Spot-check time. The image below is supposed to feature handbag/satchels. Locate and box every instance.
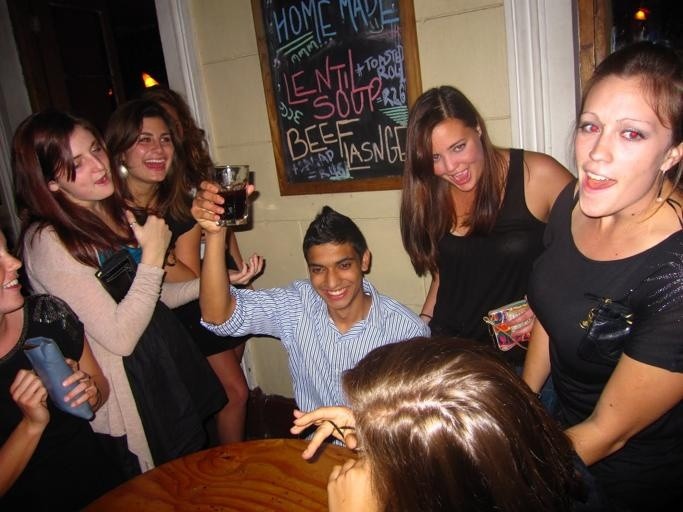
[574,289,637,369]
[23,337,96,422]
[95,247,139,304]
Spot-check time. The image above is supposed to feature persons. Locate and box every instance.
[0,228,125,512]
[104,97,202,285]
[188,180,432,453]
[9,106,264,475]
[399,85,576,378]
[517,39,683,512]
[290,337,601,512]
[143,83,257,443]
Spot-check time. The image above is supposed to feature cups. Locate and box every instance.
[210,164,253,229]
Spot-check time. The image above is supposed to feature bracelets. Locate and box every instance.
[419,313,433,320]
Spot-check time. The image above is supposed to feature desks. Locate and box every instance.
[77,436,361,512]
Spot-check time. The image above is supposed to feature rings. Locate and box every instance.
[129,222,137,228]
